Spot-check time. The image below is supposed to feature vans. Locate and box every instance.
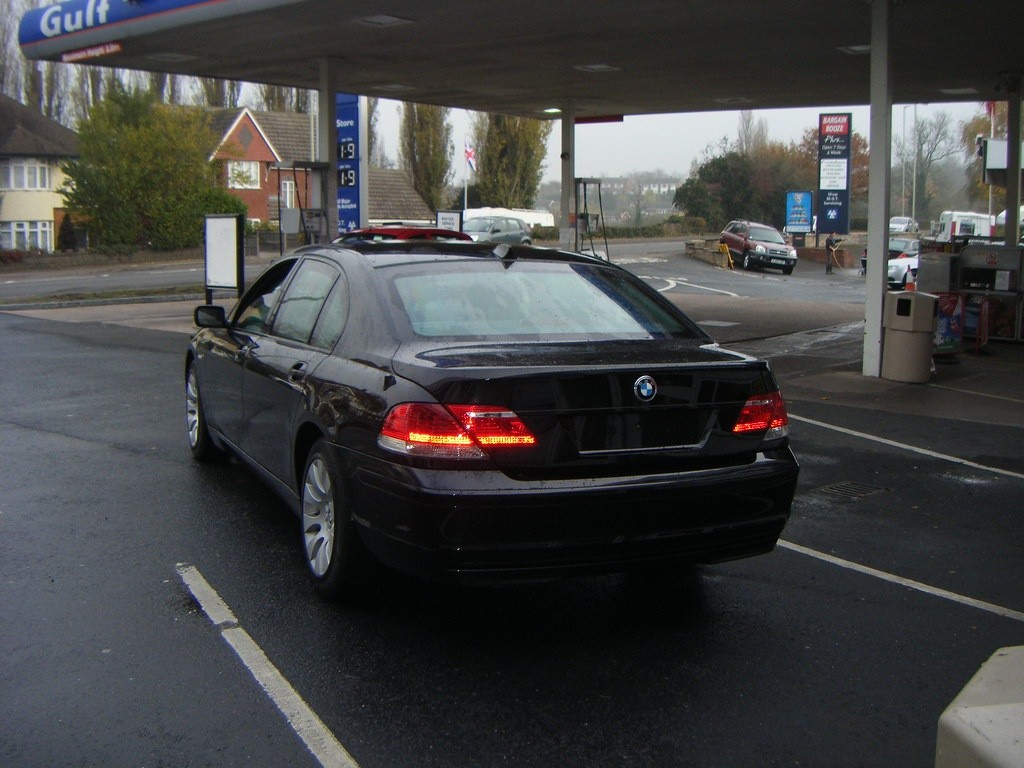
[938,210,996,244]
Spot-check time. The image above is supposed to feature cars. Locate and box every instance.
[888,217,920,235]
[179,237,803,613]
[861,235,921,277]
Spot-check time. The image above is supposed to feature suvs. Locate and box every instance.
[721,219,799,277]
[887,253,918,288]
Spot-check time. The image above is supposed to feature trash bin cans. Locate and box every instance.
[881,291,939,384]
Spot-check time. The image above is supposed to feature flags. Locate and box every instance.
[464,139,482,172]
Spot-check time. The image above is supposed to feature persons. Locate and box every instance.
[825,230,845,275]
